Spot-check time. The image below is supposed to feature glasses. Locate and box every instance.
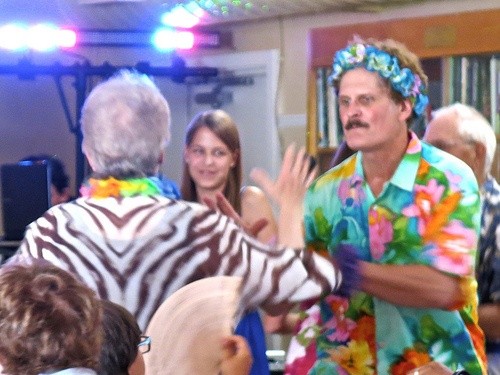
[136,336,151,354]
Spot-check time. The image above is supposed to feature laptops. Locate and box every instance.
[0,161,51,241]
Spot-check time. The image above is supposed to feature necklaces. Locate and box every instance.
[81,177,180,198]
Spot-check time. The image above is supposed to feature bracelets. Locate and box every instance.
[337,247,357,297]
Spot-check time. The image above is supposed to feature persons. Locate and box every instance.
[182,110,280,250]
[264,141,358,375]
[0,151,253,375]
[423,103,500,375]
[10,73,341,333]
[204,39,488,375]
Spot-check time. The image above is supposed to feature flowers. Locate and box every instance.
[327,42,428,117]
